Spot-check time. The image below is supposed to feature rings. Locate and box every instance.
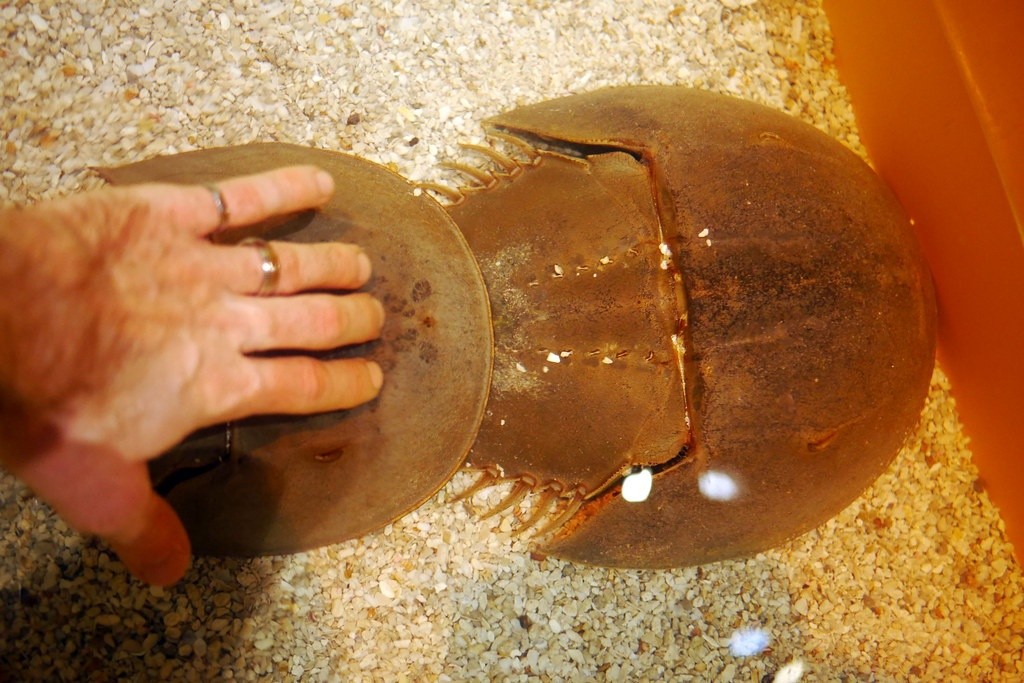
[238,237,279,296]
[202,183,226,232]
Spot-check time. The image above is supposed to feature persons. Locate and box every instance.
[0,163,386,588]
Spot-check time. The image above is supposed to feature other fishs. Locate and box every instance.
[87,144,495,560]
[414,84,930,571]
